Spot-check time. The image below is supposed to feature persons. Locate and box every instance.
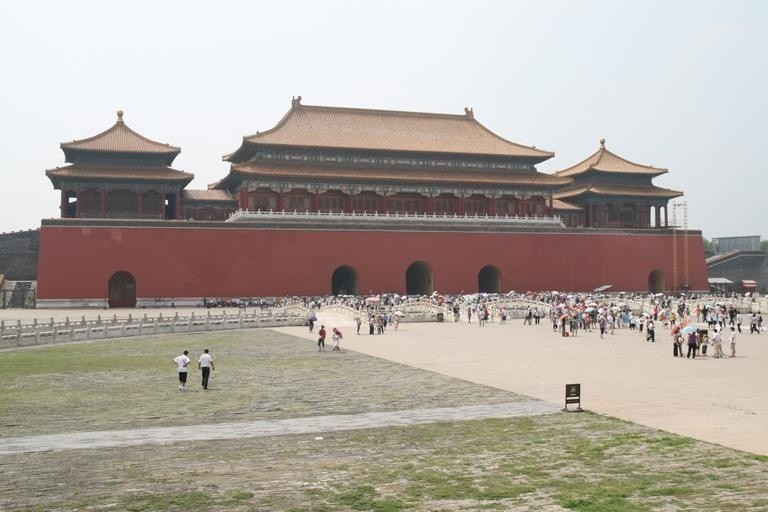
[408,290,635,340]
[325,292,402,335]
[638,290,764,358]
[198,349,214,390]
[318,326,326,350]
[205,294,324,309]
[172,350,190,391]
[332,328,343,350]
[171,297,176,309]
[307,309,316,331]
